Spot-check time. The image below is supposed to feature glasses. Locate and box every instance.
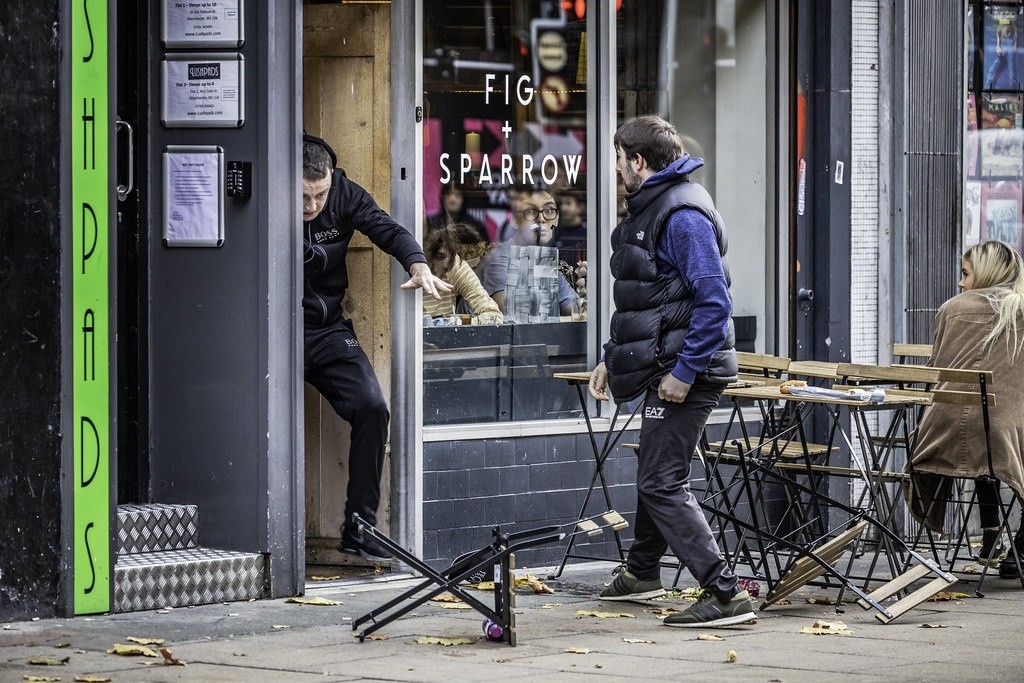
[513,207,560,221]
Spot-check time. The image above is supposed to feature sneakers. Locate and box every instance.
[340,523,395,562]
[662,587,758,628]
[598,564,667,601]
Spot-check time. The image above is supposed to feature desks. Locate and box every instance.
[551,358,928,605]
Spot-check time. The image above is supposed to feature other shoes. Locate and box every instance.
[999,559,1024,579]
[979,541,1008,564]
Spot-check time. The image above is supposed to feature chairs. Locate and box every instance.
[346,507,628,646]
[621,341,1024,625]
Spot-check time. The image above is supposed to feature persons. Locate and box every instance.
[586,115,758,628]
[549,188,587,266]
[456,180,587,320]
[901,240,1024,580]
[496,182,543,246]
[422,190,504,325]
[426,179,492,244]
[301,134,453,562]
[986,6,1020,89]
